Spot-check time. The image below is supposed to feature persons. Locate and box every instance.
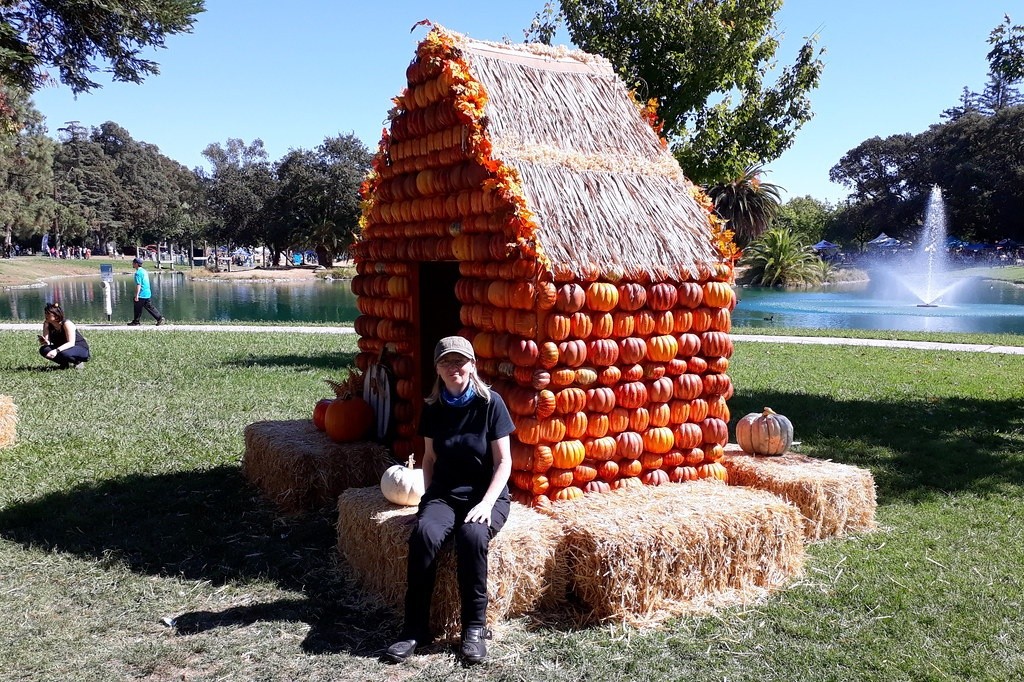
[36,302,91,370]
[0,241,92,260]
[383,335,517,666]
[127,258,165,326]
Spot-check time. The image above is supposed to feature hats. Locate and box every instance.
[435,336,475,365]
[131,258,144,266]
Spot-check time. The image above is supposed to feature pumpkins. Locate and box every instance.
[314,393,377,441]
[735,406,794,456]
[348,38,737,506]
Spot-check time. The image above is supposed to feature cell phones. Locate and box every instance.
[37,335,48,345]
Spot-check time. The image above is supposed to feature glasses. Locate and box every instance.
[437,361,471,367]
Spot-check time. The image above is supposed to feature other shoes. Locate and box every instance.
[127,320,140,326]
[459,625,488,661]
[60,361,85,370]
[156,317,165,326]
[386,637,429,663]
